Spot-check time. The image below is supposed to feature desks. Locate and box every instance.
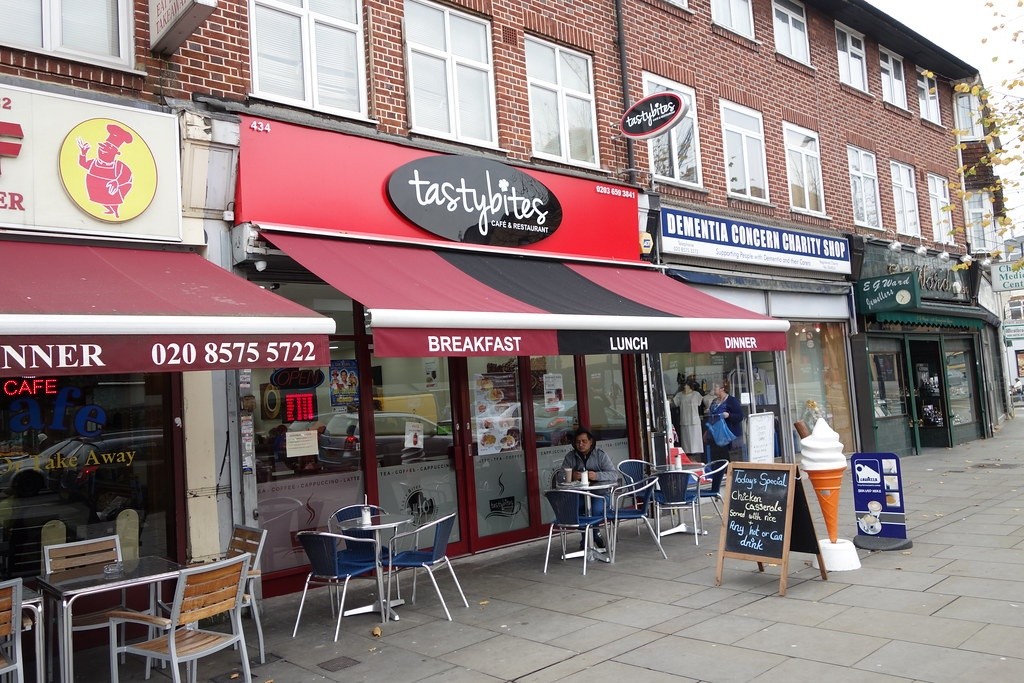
[650,463,707,537]
[35,555,188,683]
[22,585,44,683]
[557,480,616,562]
[335,513,414,622]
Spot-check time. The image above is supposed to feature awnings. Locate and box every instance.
[258,232,790,357]
[0,242,336,379]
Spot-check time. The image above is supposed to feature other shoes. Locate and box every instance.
[594,530,606,548]
[580,540,584,550]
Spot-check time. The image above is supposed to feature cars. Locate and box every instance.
[45,429,164,495]
[66,432,167,505]
[318,411,456,468]
[469,402,539,451]
[0,434,80,497]
[280,412,345,470]
[946,370,966,387]
[532,400,625,447]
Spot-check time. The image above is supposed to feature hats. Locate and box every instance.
[717,379,730,393]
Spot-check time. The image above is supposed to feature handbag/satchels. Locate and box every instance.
[705,413,737,447]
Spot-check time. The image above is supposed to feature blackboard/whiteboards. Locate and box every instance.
[747,412,775,462]
[717,461,823,564]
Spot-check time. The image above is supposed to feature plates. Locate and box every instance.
[479,380,516,450]
[859,518,882,534]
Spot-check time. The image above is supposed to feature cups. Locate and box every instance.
[563,468,572,483]
[867,501,882,520]
[866,515,877,530]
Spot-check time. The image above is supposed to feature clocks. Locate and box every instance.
[895,289,912,305]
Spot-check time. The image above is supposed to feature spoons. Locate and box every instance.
[858,518,868,532]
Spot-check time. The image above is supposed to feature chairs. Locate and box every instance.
[651,469,703,546]
[166,524,268,665]
[292,530,385,638]
[43,535,125,683]
[616,460,654,535]
[687,459,729,523]
[542,488,612,575]
[609,475,668,563]
[385,512,469,622]
[101,552,255,683]
[0,577,24,683]
[550,469,567,552]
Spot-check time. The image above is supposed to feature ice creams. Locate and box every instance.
[793,418,848,543]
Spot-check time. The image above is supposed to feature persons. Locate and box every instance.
[330,371,358,389]
[589,386,612,427]
[673,383,717,454]
[703,380,744,500]
[557,428,618,551]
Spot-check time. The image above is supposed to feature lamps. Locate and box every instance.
[928,240,950,262]
[974,249,993,270]
[904,234,928,257]
[949,242,973,265]
[862,228,902,253]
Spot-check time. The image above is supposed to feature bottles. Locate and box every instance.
[362,506,372,525]
[674,455,682,470]
